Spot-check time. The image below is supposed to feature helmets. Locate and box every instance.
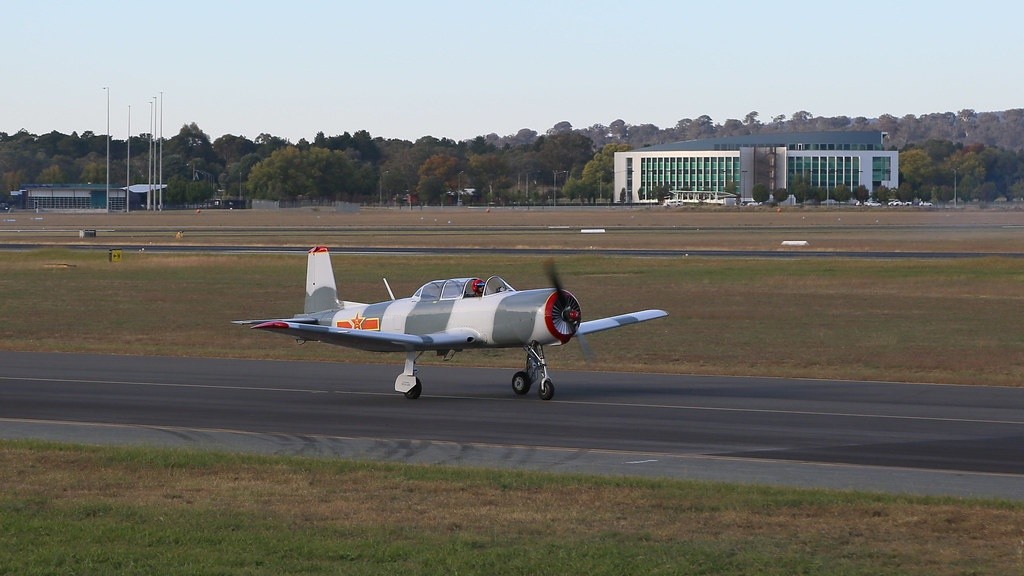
[471,280,485,293]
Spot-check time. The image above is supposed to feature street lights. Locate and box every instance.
[380,170,390,205]
[553,170,566,206]
[145,100,153,210]
[102,85,109,213]
[458,171,464,207]
[159,90,164,211]
[152,96,158,211]
[126,105,130,212]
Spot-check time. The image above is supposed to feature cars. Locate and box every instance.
[856,199,933,207]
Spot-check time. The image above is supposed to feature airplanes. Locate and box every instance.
[231,245,669,401]
